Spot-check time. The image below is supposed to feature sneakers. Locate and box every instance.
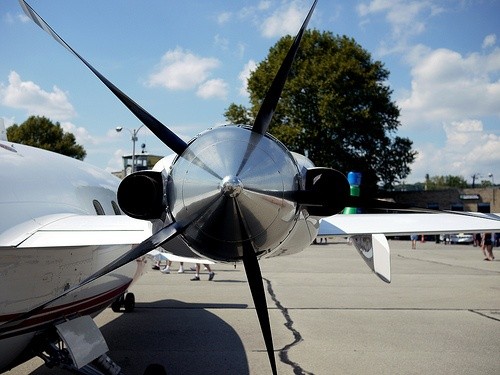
[208,272,215,281]
[191,276,200,281]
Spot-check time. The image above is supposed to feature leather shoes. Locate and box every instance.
[151,266,159,270]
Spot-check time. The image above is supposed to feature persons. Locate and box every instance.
[189,263,215,281]
[152,261,168,270]
[472,232,500,247]
[442,234,454,245]
[410,235,418,249]
[320,238,328,244]
[190,263,209,271]
[160,261,184,274]
[481,233,495,261]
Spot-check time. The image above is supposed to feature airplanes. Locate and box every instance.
[0,0,500,375]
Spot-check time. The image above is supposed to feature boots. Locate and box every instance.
[161,265,170,273]
[178,265,183,273]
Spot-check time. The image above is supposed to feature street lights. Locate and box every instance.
[115,123,145,173]
[488,172,495,206]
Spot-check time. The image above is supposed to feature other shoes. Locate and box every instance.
[484,256,494,262]
